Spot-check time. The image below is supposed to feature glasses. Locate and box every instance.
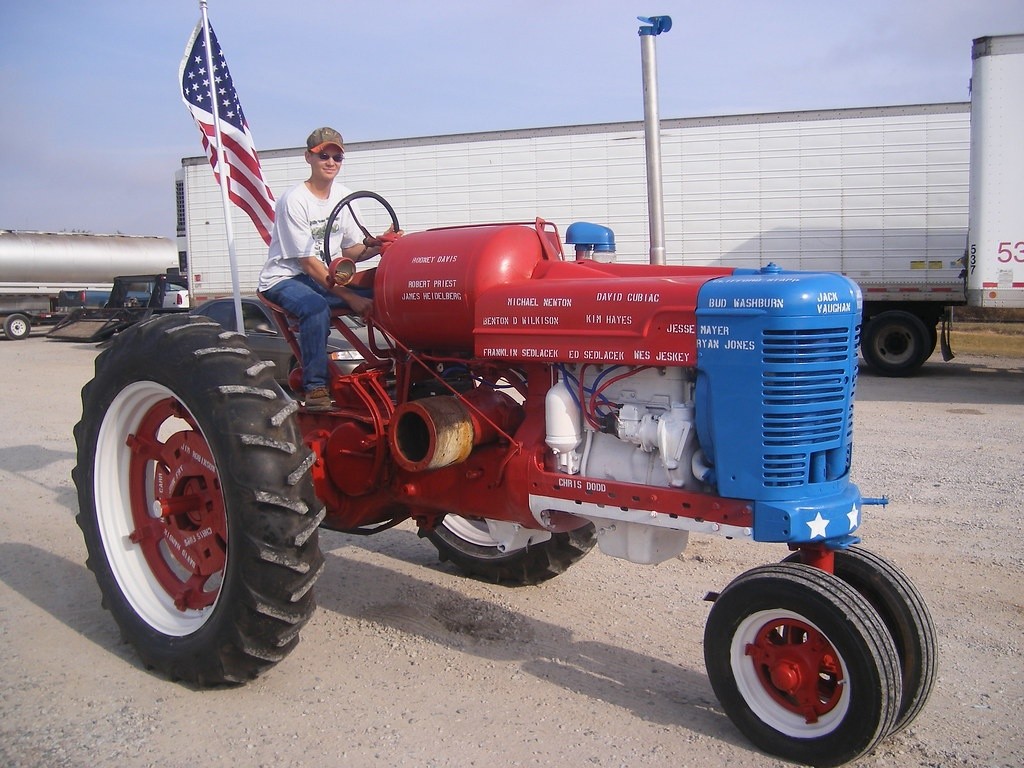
[316,153,346,162]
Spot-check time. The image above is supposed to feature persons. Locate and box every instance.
[258,127,404,411]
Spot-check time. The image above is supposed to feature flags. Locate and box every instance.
[179,16,275,247]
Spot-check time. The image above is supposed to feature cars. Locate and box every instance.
[149,281,189,310]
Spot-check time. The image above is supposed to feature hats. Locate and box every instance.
[306,126,347,155]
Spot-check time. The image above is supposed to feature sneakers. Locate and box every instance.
[304,385,333,408]
